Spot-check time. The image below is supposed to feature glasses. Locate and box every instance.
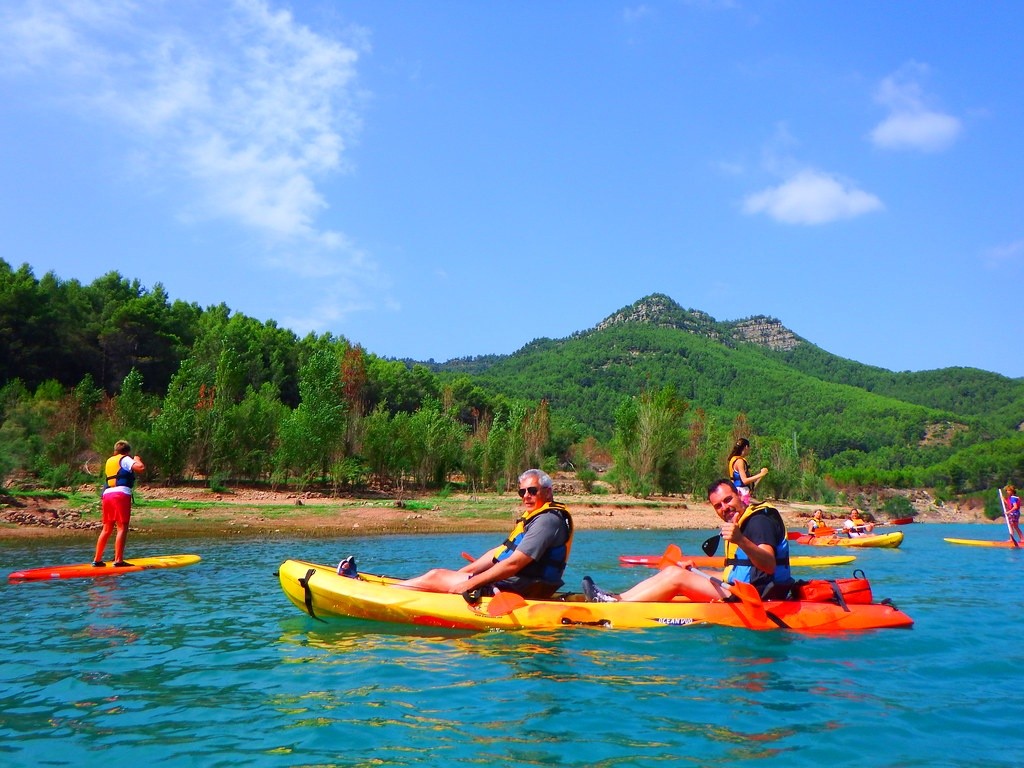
[518,486,546,498]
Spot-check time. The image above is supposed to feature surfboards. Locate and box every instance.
[7,554,202,583]
[621,555,855,571]
[943,538,1024,549]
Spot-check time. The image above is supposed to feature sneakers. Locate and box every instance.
[582,575,619,602]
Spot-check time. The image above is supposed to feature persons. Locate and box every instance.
[336,469,575,599]
[92,440,144,567]
[583,477,790,610]
[1001,485,1024,543]
[841,509,876,539]
[729,437,769,498]
[807,509,839,540]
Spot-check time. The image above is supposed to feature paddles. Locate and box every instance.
[657,544,765,609]
[787,531,811,541]
[815,517,912,536]
[701,471,766,557]
[461,552,529,617]
[998,487,1019,547]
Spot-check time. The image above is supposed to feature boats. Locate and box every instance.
[275,556,916,634]
[788,529,904,548]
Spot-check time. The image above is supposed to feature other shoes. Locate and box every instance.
[91,561,105,567]
[113,561,135,566]
[336,556,358,579]
